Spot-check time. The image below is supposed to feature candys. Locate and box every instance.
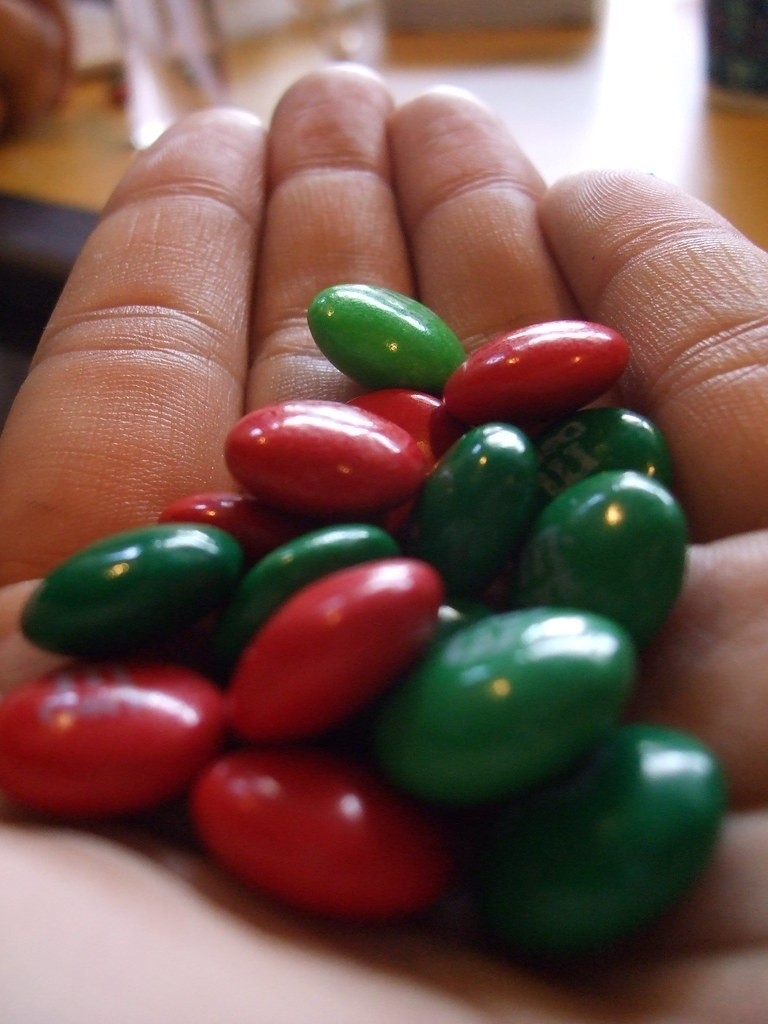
[5,286,728,959]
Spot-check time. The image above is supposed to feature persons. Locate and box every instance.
[0,66,768,1024]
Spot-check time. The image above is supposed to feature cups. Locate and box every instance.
[114,0,392,154]
